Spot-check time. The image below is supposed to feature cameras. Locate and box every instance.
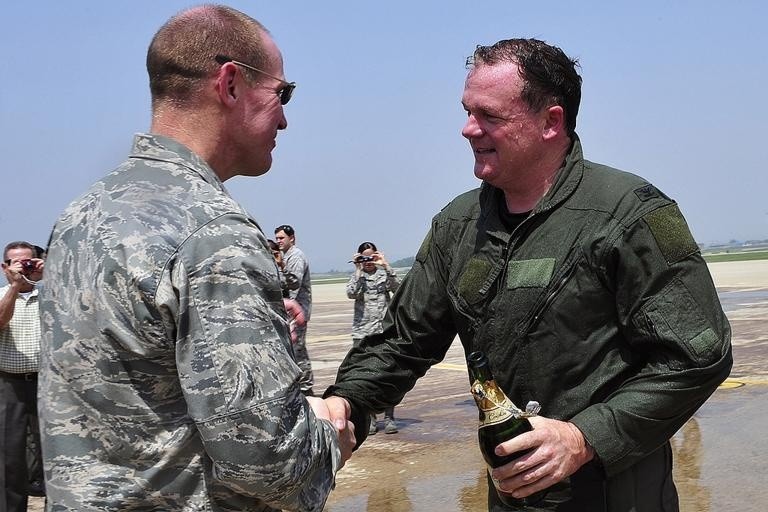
[358,256,373,263]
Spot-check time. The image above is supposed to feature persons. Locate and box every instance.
[264,239,301,301]
[325,40,733,511]
[274,225,314,399]
[37,4,357,512]
[0,240,45,512]
[30,245,46,281]
[345,243,402,434]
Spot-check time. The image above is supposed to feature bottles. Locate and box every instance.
[467,352,551,509]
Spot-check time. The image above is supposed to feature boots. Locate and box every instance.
[383,408,397,434]
[369,412,377,435]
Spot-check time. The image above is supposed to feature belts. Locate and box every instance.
[24,373,33,382]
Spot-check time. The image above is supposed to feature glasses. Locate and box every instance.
[214,54,296,105]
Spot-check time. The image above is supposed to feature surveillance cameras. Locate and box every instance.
[19,259,37,274]
